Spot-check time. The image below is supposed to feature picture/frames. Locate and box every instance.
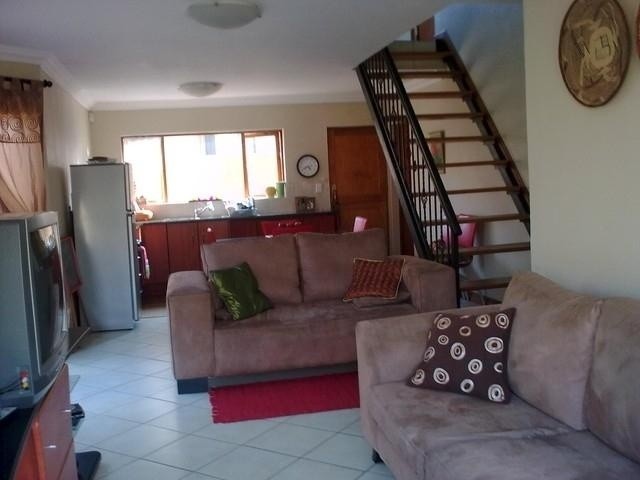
[294,196,316,214]
[424,130,447,175]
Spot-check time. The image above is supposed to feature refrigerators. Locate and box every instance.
[69,161,144,331]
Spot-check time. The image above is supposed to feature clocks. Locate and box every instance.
[296,153,321,178]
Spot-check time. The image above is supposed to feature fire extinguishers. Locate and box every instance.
[136,239,150,280]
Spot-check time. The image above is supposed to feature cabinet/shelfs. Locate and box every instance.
[200,217,256,271]
[135,222,200,303]
[256,212,334,238]
[1,325,95,480]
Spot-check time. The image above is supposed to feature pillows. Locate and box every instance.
[209,261,274,319]
[344,258,405,299]
[407,307,515,405]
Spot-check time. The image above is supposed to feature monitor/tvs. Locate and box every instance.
[0,210,70,408]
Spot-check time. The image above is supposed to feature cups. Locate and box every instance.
[276,181,284,197]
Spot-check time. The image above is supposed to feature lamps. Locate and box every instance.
[186,1,261,29]
[178,82,221,98]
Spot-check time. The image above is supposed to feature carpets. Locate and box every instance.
[210,374,358,422]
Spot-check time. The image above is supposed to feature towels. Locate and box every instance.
[136,246,151,280]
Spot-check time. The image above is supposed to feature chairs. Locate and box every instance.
[352,215,368,233]
[432,213,477,302]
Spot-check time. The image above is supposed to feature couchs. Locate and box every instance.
[355,270,638,479]
[165,228,457,393]
